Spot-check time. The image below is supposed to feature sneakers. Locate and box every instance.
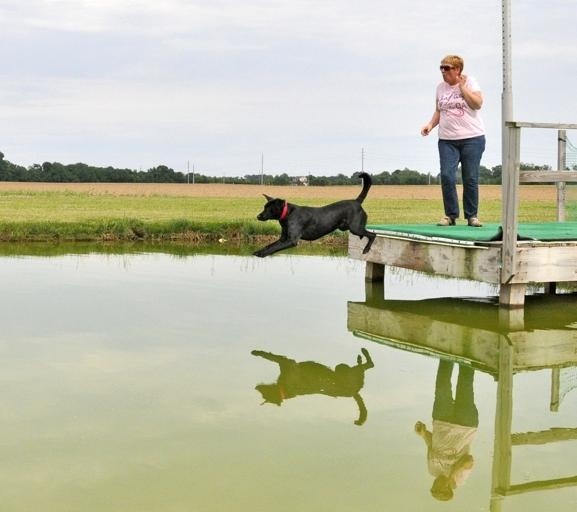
[436,216,456,226]
[468,218,482,227]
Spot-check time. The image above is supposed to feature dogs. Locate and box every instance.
[250,348,374,426]
[251,172,377,258]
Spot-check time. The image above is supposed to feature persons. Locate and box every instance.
[421,55,486,227]
[414,357,479,503]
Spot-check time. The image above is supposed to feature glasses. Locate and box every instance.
[440,65,454,71]
